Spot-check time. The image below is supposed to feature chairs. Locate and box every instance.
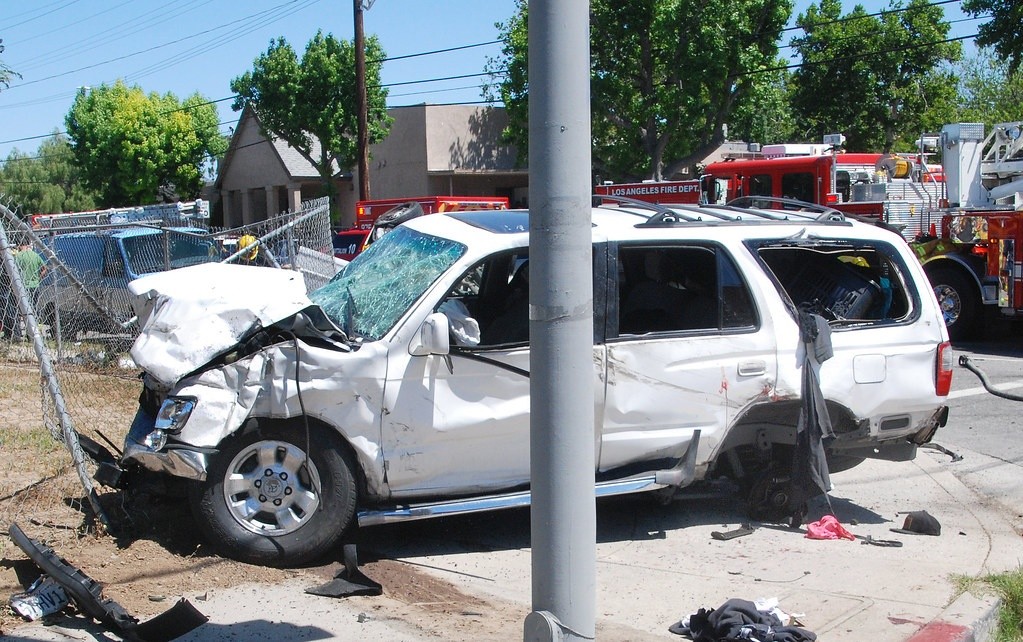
[481,249,719,346]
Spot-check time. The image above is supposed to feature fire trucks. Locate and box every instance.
[330,194,509,268]
[593,118,1023,339]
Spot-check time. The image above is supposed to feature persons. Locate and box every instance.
[14,235,46,343]
[1002,240,1014,307]
[222,235,271,267]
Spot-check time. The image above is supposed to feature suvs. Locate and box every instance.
[117,198,962,566]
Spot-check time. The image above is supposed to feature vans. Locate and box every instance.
[31,228,224,341]
